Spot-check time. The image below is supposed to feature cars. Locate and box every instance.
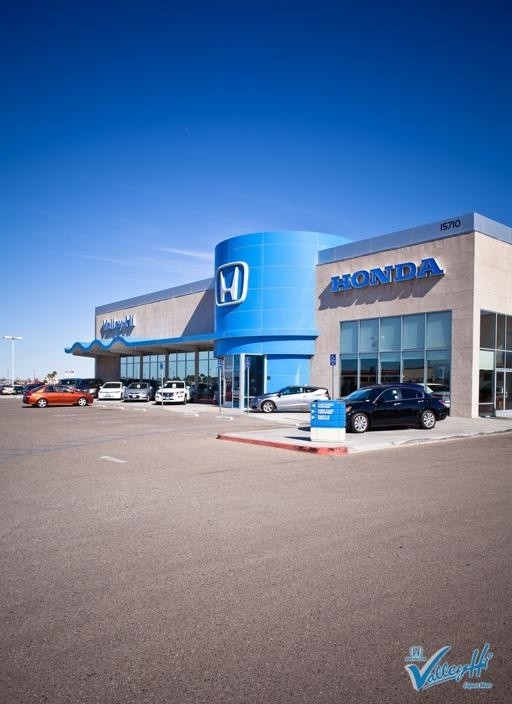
[417,382,451,407]
[193,383,214,397]
[22,383,94,408]
[332,384,448,434]
[96,381,123,400]
[0,377,104,399]
[153,380,193,406]
[249,384,331,413]
[124,379,154,402]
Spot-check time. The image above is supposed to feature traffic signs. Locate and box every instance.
[310,398,346,428]
